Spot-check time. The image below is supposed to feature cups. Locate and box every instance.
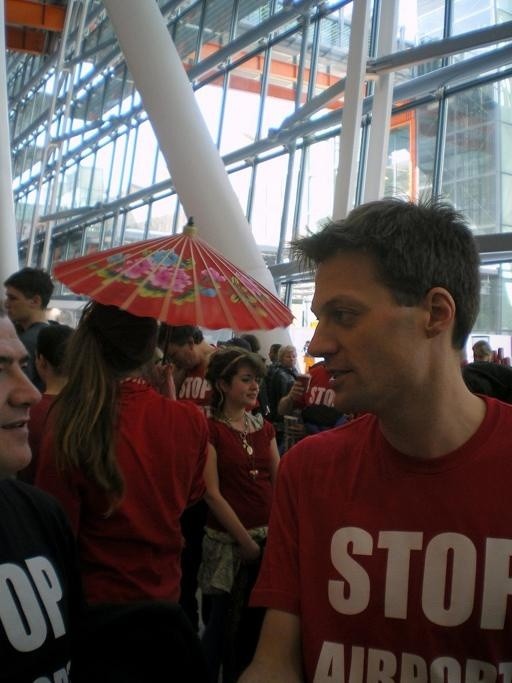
[295,373,312,392]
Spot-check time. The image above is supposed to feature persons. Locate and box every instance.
[1,266,360,683]
[242,200,512,683]
